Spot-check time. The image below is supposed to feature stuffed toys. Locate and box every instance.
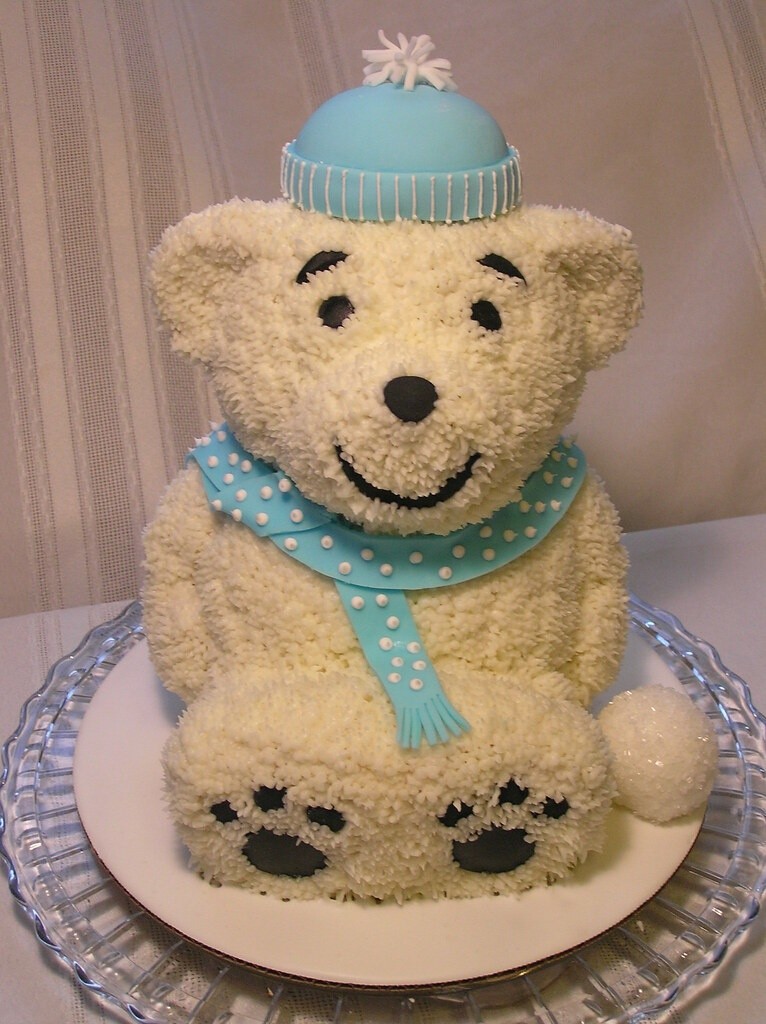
[142,30,643,904]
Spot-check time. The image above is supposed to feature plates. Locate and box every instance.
[0,594,766,1024]
